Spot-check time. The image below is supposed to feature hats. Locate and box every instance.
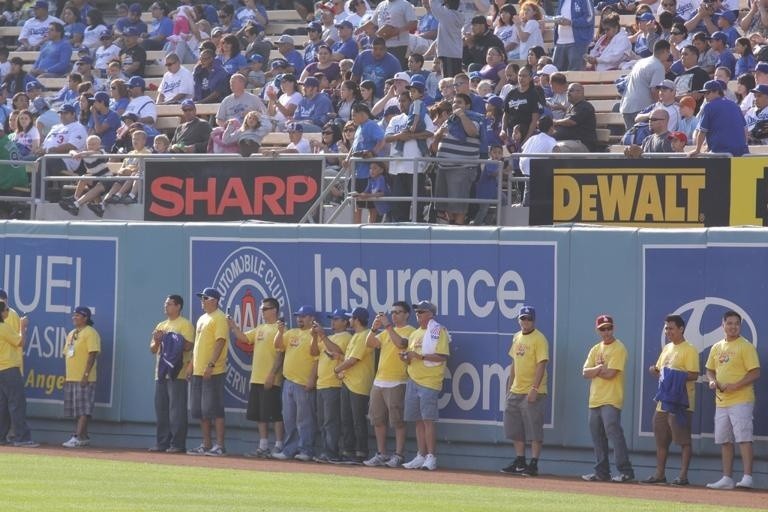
[412,300,437,314]
[195,288,221,300]
[596,315,613,328]
[0,0,768,132]
[327,308,350,320]
[73,306,92,317]
[292,304,315,317]
[668,132,686,144]
[519,306,535,318]
[344,307,369,319]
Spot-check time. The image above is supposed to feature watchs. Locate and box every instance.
[83,373,90,377]
[207,363,215,368]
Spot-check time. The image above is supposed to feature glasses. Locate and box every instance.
[201,295,214,300]
[520,316,532,320]
[2,308,10,312]
[71,313,86,318]
[414,309,430,314]
[390,309,407,315]
[261,306,273,311]
[599,325,613,331]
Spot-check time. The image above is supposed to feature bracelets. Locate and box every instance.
[370,328,377,333]
[532,385,539,390]
[334,367,337,374]
[421,354,425,361]
[386,324,393,328]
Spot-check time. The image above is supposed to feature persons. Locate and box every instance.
[309,308,353,463]
[640,314,700,486]
[330,307,377,465]
[705,310,761,490]
[149,294,195,453]
[582,315,635,483]
[0,289,40,446]
[62,307,101,447]
[362,300,416,466]
[273,306,322,461]
[192,288,228,456]
[500,305,549,477]
[226,296,288,458]
[0,289,24,376]
[1,1,766,221]
[399,300,449,471]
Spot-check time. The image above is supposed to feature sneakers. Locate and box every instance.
[520,465,538,478]
[500,463,527,474]
[736,476,755,489]
[59,195,137,218]
[150,441,226,456]
[14,437,40,448]
[243,446,437,471]
[582,470,611,482]
[61,435,91,447]
[639,474,667,486]
[0,438,6,445]
[671,477,689,486]
[706,477,734,488]
[611,472,634,483]
[330,192,346,205]
